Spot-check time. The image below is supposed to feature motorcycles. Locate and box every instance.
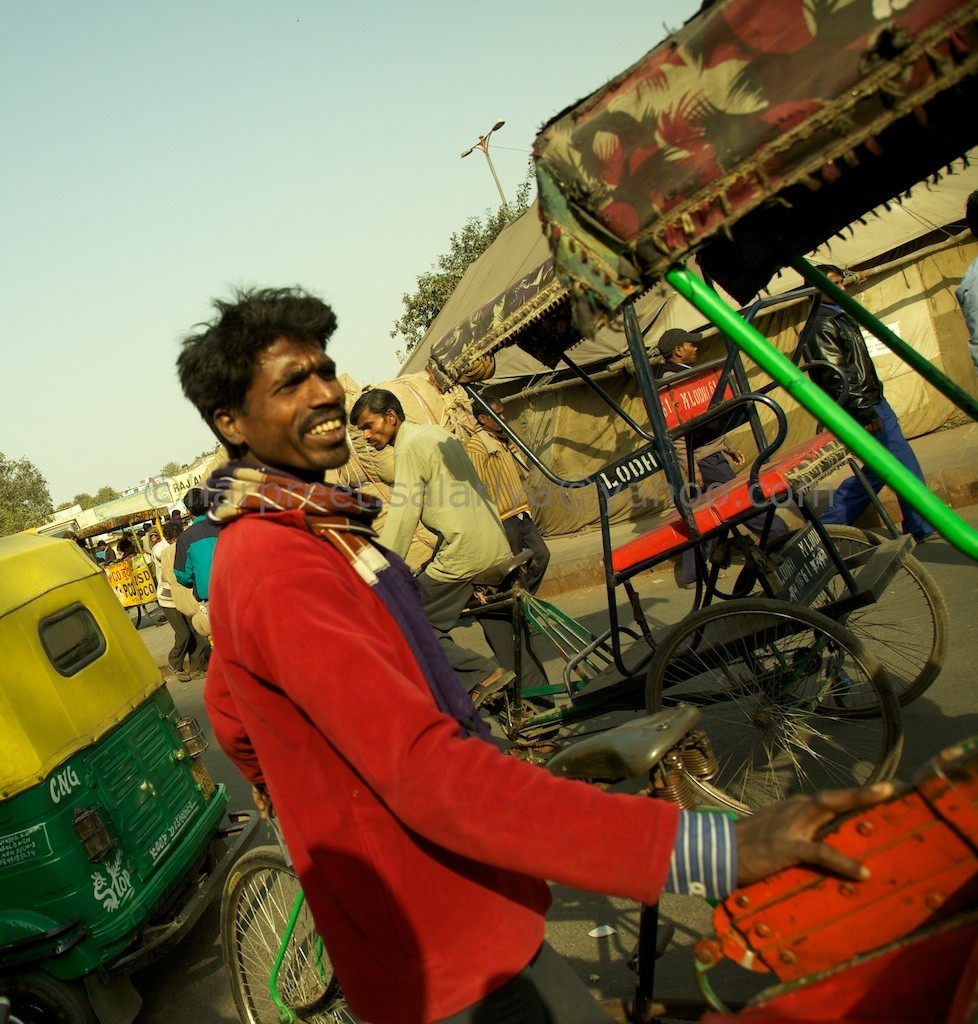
[1,535,260,1024]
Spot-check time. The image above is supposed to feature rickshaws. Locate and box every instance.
[223,0,978,1024]
[77,507,170,630]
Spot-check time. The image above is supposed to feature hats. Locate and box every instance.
[658,328,703,355]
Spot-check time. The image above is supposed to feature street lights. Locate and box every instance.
[460,120,511,226]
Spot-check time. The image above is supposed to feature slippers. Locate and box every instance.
[189,671,208,679]
[471,671,517,709]
[168,664,192,681]
[505,740,562,761]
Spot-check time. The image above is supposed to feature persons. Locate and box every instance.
[954,191,978,371]
[464,395,550,636]
[119,532,135,554]
[800,264,942,544]
[656,328,790,589]
[141,517,164,552]
[170,510,181,524]
[97,541,116,561]
[174,488,220,602]
[152,522,211,681]
[178,289,893,1024]
[350,388,560,765]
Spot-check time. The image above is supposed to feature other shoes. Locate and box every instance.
[719,573,727,578]
[685,580,698,589]
[918,530,940,543]
[527,623,545,636]
